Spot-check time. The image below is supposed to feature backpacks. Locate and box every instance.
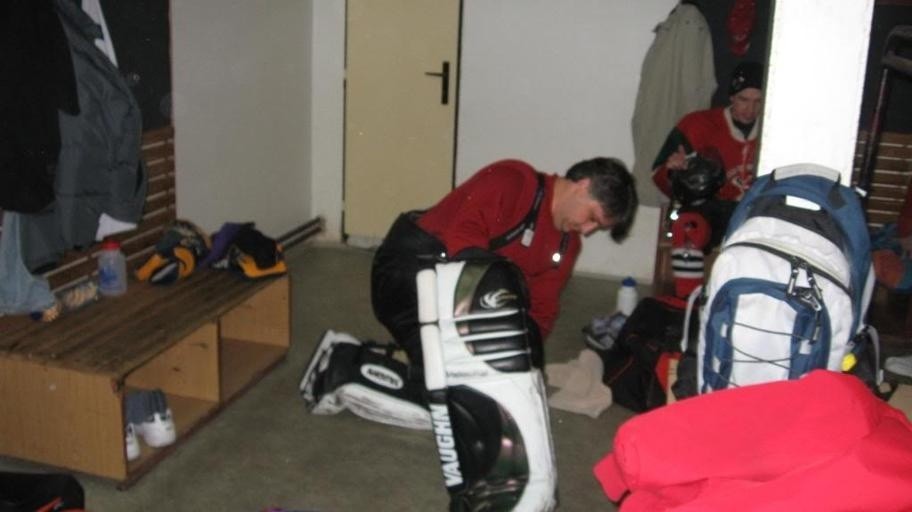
[671,163,883,402]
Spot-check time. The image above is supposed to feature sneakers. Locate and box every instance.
[124,388,176,461]
[300,330,360,415]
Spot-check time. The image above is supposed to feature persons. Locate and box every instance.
[299,158,637,430]
[650,68,765,300]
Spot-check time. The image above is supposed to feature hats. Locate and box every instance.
[728,61,764,96]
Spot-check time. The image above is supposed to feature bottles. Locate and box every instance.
[617,277,638,315]
[98,242,127,298]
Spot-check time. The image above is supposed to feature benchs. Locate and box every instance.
[1,126,294,493]
[656,117,909,348]
